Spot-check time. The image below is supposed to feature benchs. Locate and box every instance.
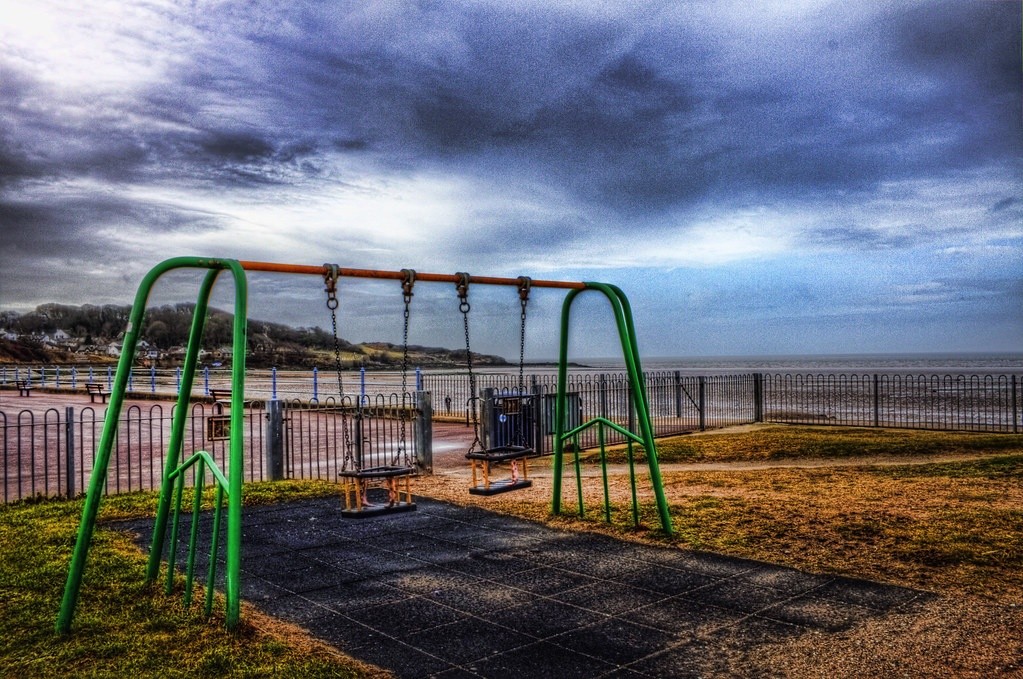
[208,388,251,417]
[85,383,112,404]
[15,380,37,397]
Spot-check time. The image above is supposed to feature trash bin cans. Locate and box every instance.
[493,390,535,458]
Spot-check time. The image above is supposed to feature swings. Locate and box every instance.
[320,262,420,520]
[454,270,535,498]
